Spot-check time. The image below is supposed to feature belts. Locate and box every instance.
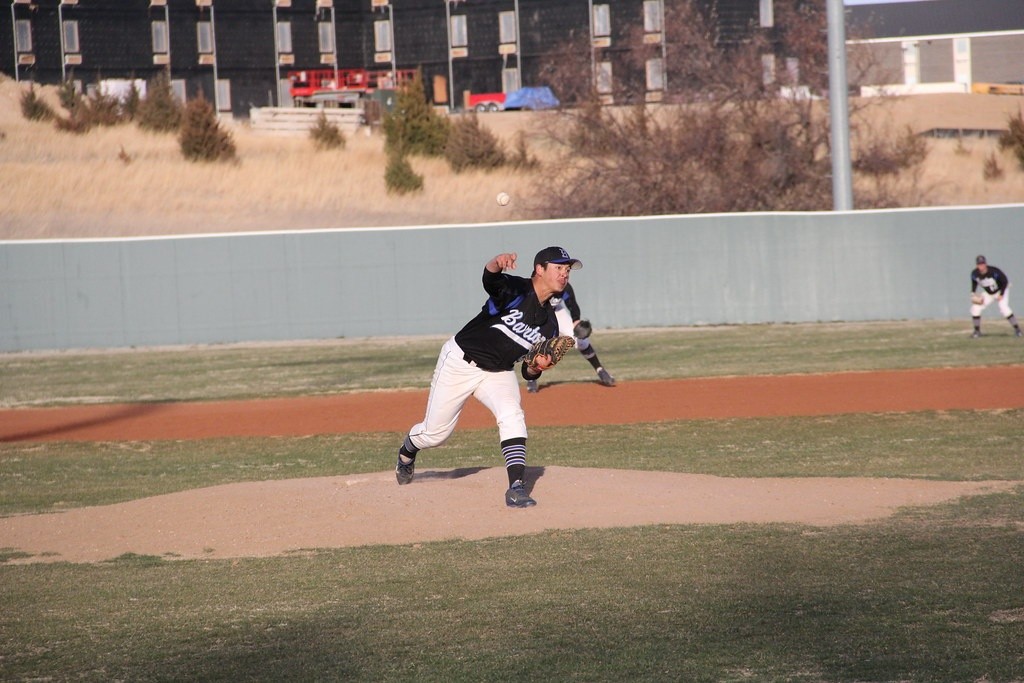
[463,354,486,369]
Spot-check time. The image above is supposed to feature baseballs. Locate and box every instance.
[495,192,510,206]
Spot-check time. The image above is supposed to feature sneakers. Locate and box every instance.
[395,444,416,485]
[505,485,537,508]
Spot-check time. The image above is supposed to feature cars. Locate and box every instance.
[989,82,1024,96]
[693,84,729,104]
[753,71,861,100]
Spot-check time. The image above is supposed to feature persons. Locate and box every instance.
[970,255,1024,341]
[526,280,617,392]
[396,247,583,508]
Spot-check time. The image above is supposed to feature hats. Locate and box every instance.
[977,256,985,263]
[534,247,583,270]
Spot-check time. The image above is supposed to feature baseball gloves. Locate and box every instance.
[971,294,984,305]
[522,335,576,373]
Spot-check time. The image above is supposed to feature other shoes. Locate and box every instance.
[527,381,538,392]
[599,369,615,386]
[1016,330,1022,336]
[971,330,981,338]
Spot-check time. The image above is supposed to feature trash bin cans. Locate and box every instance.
[364,99,380,124]
[373,89,394,116]
